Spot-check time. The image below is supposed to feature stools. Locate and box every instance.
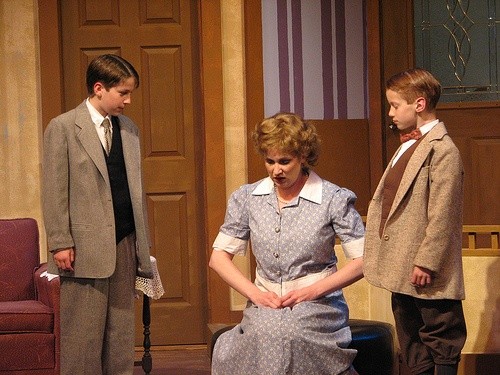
[211,319,400,375]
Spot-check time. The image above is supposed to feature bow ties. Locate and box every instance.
[399,129,421,142]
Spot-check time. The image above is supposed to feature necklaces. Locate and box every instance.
[275,175,308,204]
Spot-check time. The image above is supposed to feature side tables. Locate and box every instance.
[134,255,165,375]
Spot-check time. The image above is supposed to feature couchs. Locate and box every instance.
[0,218,61,375]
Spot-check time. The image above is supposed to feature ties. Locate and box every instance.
[101,119,112,155]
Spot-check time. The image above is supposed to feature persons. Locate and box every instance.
[363,70,468,375]
[41,54,152,375]
[209,113,365,375]
